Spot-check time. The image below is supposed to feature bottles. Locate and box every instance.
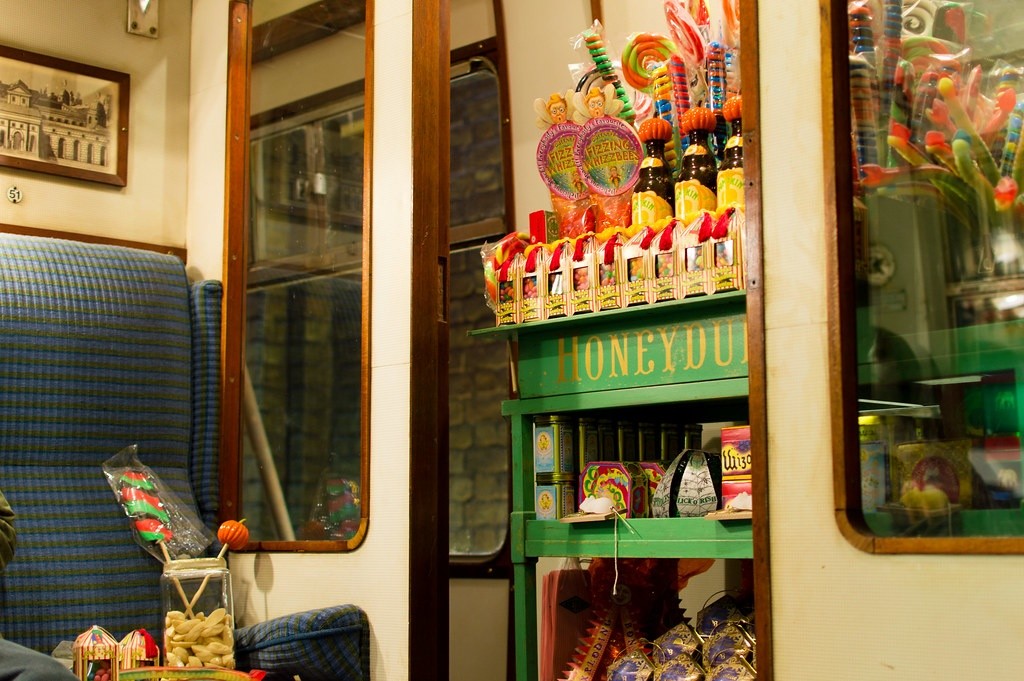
[675,130,717,220]
[717,118,745,214]
[159,557,236,671]
[630,138,675,224]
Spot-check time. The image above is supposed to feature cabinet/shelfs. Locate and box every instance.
[470,291,758,680]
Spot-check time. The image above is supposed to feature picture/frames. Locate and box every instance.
[0,44,131,187]
